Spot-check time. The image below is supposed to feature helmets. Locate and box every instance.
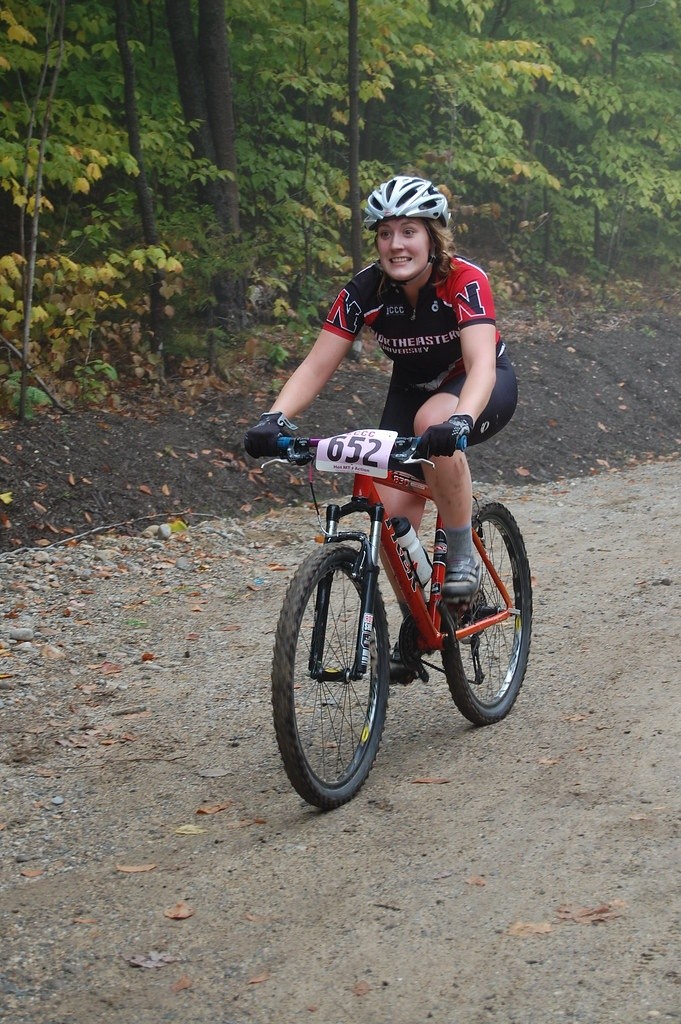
[363,176,449,231]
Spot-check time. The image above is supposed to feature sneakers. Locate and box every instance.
[388,642,406,679]
[442,552,480,597]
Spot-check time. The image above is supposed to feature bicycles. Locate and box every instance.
[242,432,534,809]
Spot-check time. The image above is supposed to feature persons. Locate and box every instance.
[241,177,517,683]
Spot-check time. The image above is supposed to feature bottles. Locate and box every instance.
[391,515,433,587]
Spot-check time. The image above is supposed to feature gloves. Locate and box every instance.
[244,412,298,459]
[413,414,473,460]
